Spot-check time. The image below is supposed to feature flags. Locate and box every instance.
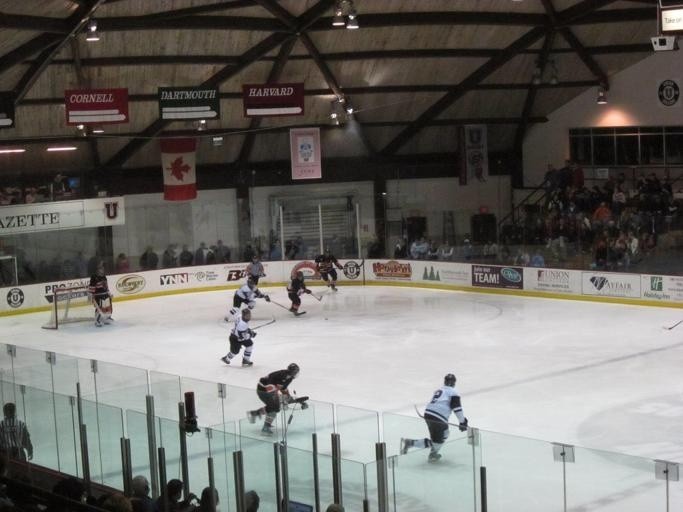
[160,137,198,201]
[291,127,322,179]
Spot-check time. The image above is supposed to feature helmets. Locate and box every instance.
[289,363,299,375]
[444,374,456,385]
[322,247,331,253]
[240,309,251,318]
[95,265,105,276]
[246,277,256,289]
[295,270,304,279]
[253,254,261,260]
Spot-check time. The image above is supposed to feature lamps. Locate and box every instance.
[86,19,100,42]
[347,0,360,30]
[331,0,346,27]
[596,87,607,105]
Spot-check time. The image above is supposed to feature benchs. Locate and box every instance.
[0,476,109,512]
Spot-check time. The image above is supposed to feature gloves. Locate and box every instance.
[338,265,343,270]
[88,292,95,303]
[458,419,468,432]
[307,289,312,294]
[265,294,270,302]
[250,331,257,338]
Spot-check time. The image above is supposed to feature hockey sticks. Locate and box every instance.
[332,258,365,270]
[414,402,459,426]
[270,300,306,315]
[662,320,683,331]
[310,293,323,301]
[251,313,275,330]
[81,280,111,321]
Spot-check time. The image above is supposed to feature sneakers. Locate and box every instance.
[223,317,230,321]
[327,280,337,290]
[250,410,259,423]
[260,426,274,434]
[241,358,253,366]
[427,453,441,459]
[96,318,114,327]
[220,356,232,364]
[402,437,412,455]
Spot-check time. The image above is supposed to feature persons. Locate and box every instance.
[0,171,103,205]
[247,364,301,433]
[323,234,352,259]
[245,489,260,511]
[395,159,683,272]
[18,231,308,279]
[287,272,312,316]
[399,373,469,461]
[315,248,343,291]
[221,308,256,365]
[225,278,269,321]
[52,475,220,511]
[89,269,113,327]
[1,403,34,461]
[4,469,34,510]
